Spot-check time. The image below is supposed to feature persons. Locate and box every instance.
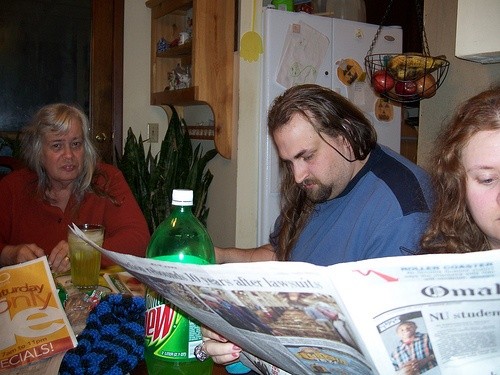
[202,86,500,365]
[0,103,152,273]
[215,83,438,266]
[390,320,438,375]
[142,275,345,340]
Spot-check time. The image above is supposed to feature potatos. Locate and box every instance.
[387,73,437,97]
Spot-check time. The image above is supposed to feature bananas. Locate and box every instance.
[389,54,438,81]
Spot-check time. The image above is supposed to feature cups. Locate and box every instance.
[178,32,189,46]
[68,224,104,291]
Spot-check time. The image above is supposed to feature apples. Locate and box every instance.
[372,70,419,96]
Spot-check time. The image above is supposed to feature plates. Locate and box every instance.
[63,285,113,314]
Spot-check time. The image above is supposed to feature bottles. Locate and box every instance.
[144,189,217,375]
[185,65,191,87]
[175,63,183,85]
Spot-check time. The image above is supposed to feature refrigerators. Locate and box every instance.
[258,9,403,248]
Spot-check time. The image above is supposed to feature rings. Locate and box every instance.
[201,341,207,355]
[65,255,70,261]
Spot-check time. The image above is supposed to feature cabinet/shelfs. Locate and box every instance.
[145,0,235,159]
